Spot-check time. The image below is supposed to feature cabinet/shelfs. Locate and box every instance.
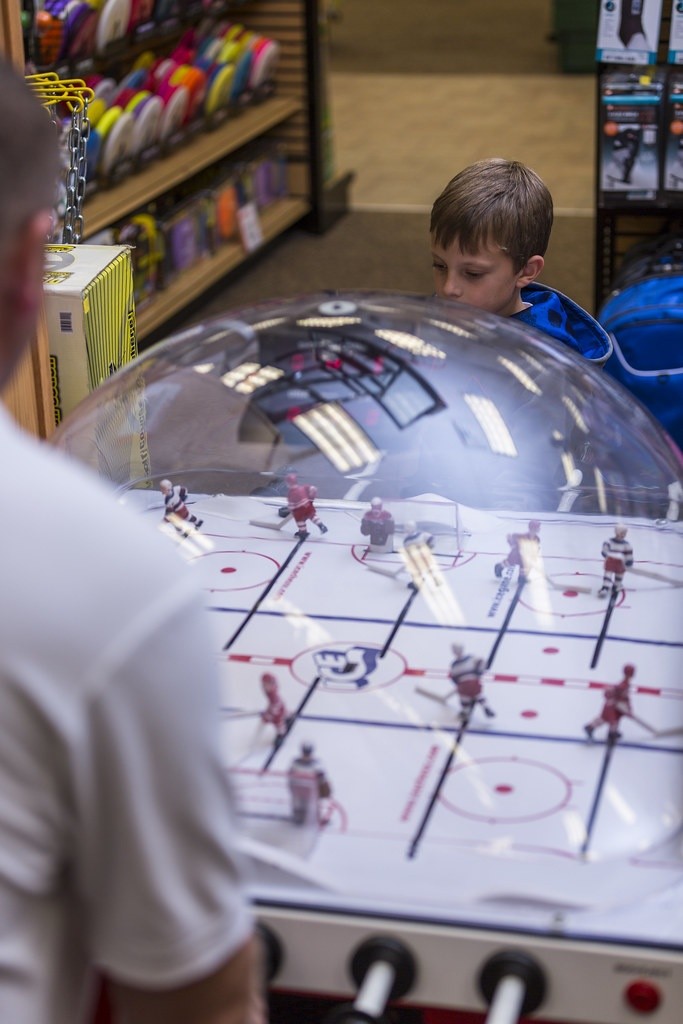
[0,0,325,344]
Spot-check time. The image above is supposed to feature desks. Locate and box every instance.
[118,487,682,1023]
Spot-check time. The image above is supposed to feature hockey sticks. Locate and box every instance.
[544,574,593,595]
[248,513,293,531]
[148,501,196,511]
[227,721,266,770]
[413,686,458,706]
[626,565,683,587]
[326,508,362,523]
[368,563,409,579]
[629,714,683,739]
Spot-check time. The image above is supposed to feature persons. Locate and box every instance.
[495,520,540,584]
[596,524,635,598]
[285,473,327,539]
[584,664,635,739]
[359,496,395,544]
[287,740,329,824]
[403,520,436,588]
[0,63,264,1024]
[159,479,203,538]
[260,675,288,734]
[427,157,613,369]
[447,644,494,720]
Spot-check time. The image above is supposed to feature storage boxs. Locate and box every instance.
[41,243,154,490]
